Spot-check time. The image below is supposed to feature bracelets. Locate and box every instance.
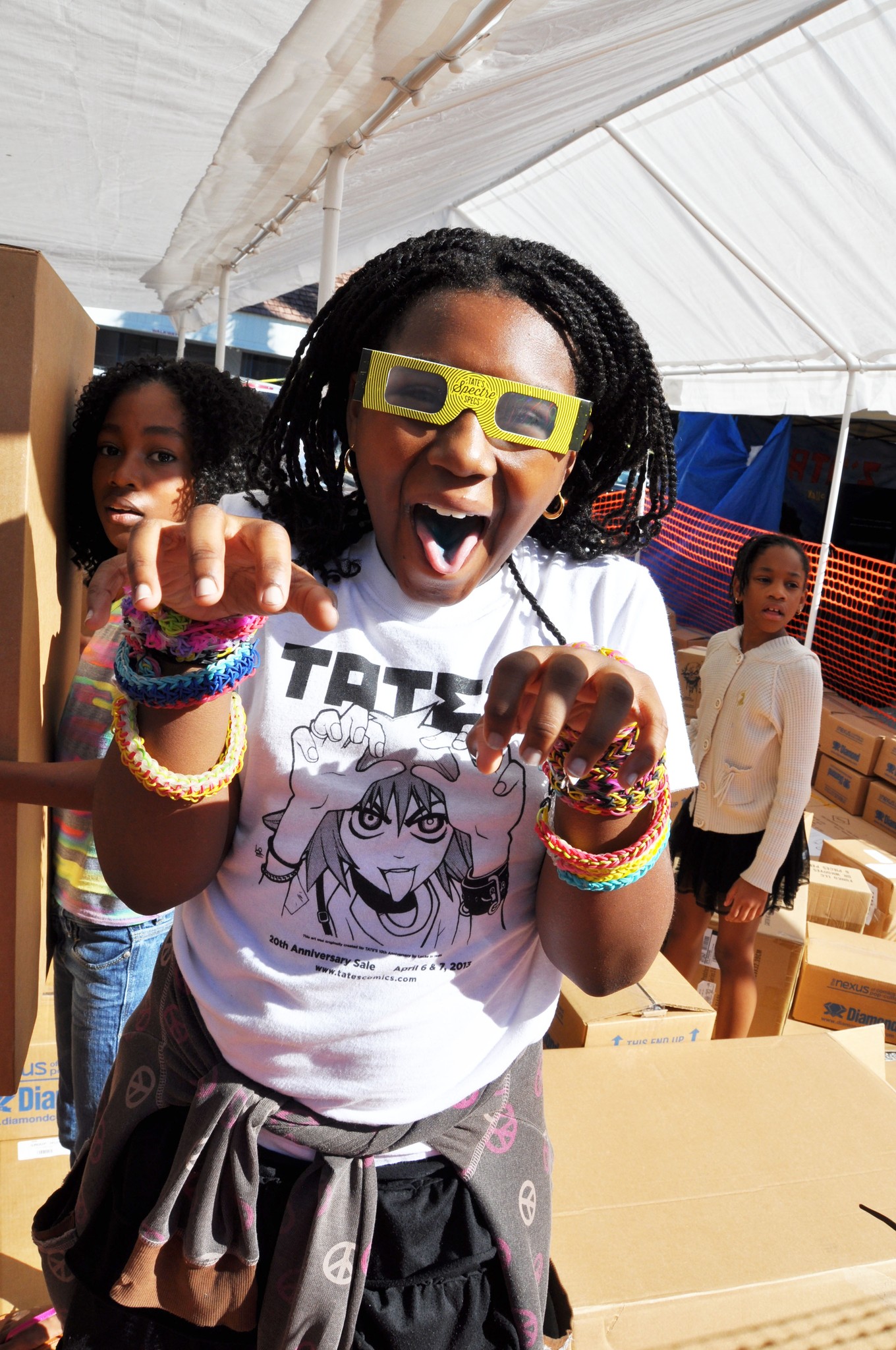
[117,589,271,806]
[535,640,673,891]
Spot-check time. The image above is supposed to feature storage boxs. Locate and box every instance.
[0,242,896,1350]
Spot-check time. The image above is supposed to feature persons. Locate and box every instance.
[660,532,823,1040]
[33,228,700,1349]
[0,356,273,1168]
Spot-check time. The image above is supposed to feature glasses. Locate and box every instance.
[352,348,592,454]
